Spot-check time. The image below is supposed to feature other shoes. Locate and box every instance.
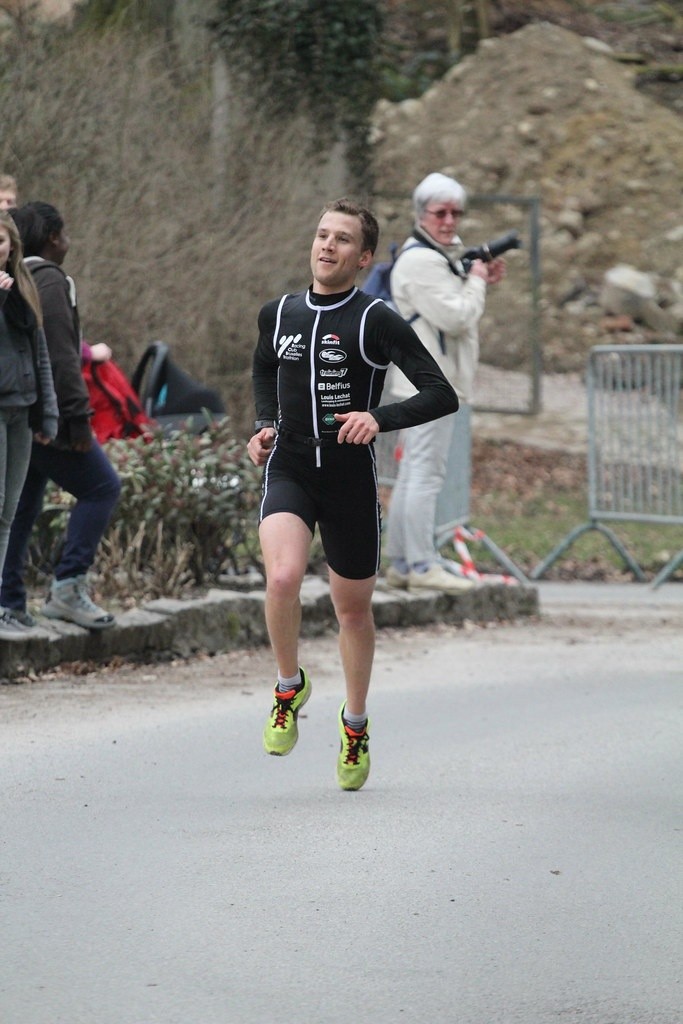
[385,563,409,588]
[408,563,474,591]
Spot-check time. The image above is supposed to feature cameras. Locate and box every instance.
[460,232,521,275]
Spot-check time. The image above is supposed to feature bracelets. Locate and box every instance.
[254,420,277,434]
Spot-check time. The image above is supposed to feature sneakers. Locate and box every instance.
[336,699,370,790]
[41,574,115,628]
[262,667,312,755]
[0,608,38,630]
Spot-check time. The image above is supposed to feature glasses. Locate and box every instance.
[426,208,463,218]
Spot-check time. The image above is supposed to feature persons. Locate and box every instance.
[367,172,508,596]
[247,196,459,792]
[0,172,160,642]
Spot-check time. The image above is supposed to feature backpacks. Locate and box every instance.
[361,243,460,323]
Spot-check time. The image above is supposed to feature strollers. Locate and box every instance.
[91,341,224,440]
